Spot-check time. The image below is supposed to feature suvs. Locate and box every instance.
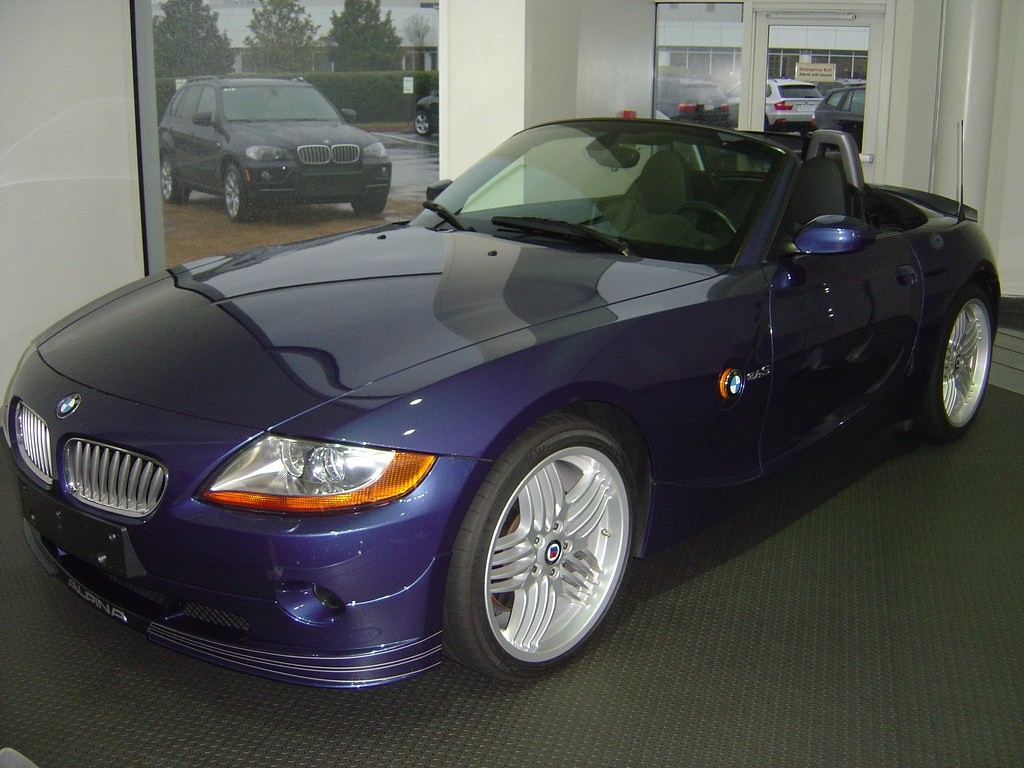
[726,78,823,133]
[157,75,392,222]
[656,77,729,127]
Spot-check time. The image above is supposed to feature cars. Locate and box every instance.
[811,79,866,97]
[810,86,868,151]
[413,86,438,139]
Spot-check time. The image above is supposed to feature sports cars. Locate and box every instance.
[1,111,1001,692]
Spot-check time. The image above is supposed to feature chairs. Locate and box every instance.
[599,148,698,239]
[789,155,854,247]
